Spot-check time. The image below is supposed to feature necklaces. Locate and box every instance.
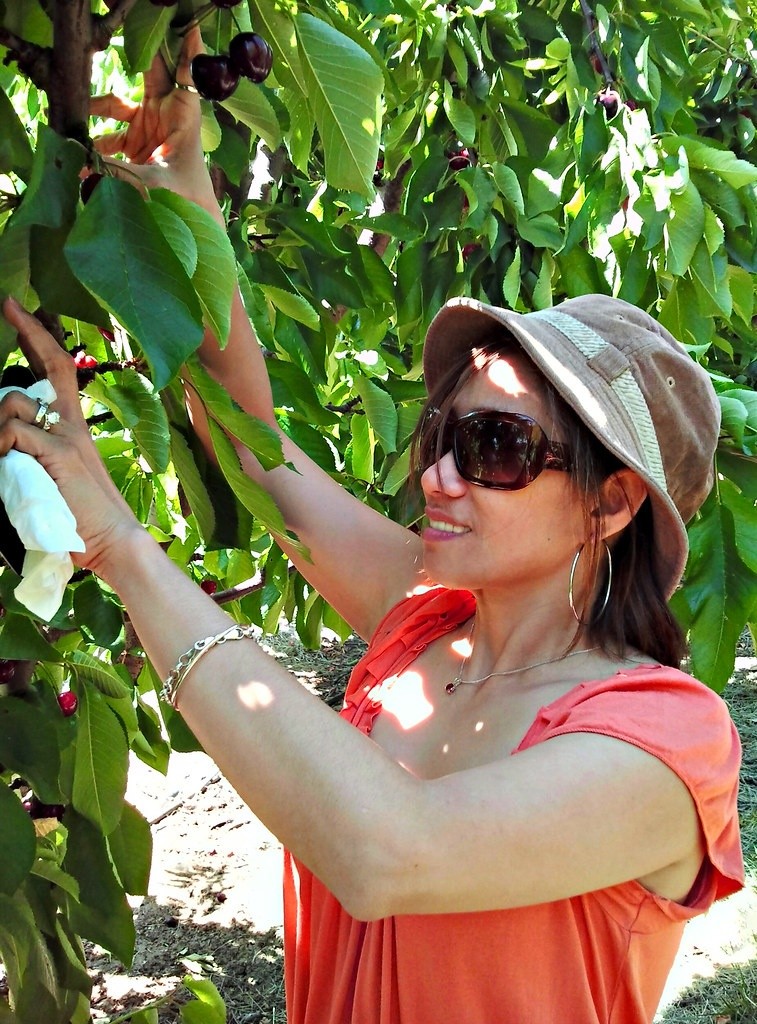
[445,621,602,694]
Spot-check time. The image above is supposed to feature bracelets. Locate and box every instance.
[158,624,264,712]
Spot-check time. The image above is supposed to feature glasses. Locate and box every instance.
[419,407,613,492]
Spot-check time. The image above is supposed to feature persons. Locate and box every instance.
[0,16,746,1024]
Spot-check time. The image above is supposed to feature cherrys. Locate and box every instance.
[0,4,646,821]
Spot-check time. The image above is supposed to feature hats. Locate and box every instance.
[423,294,721,603]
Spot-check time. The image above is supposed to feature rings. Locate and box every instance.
[175,81,199,94]
[31,398,58,432]
